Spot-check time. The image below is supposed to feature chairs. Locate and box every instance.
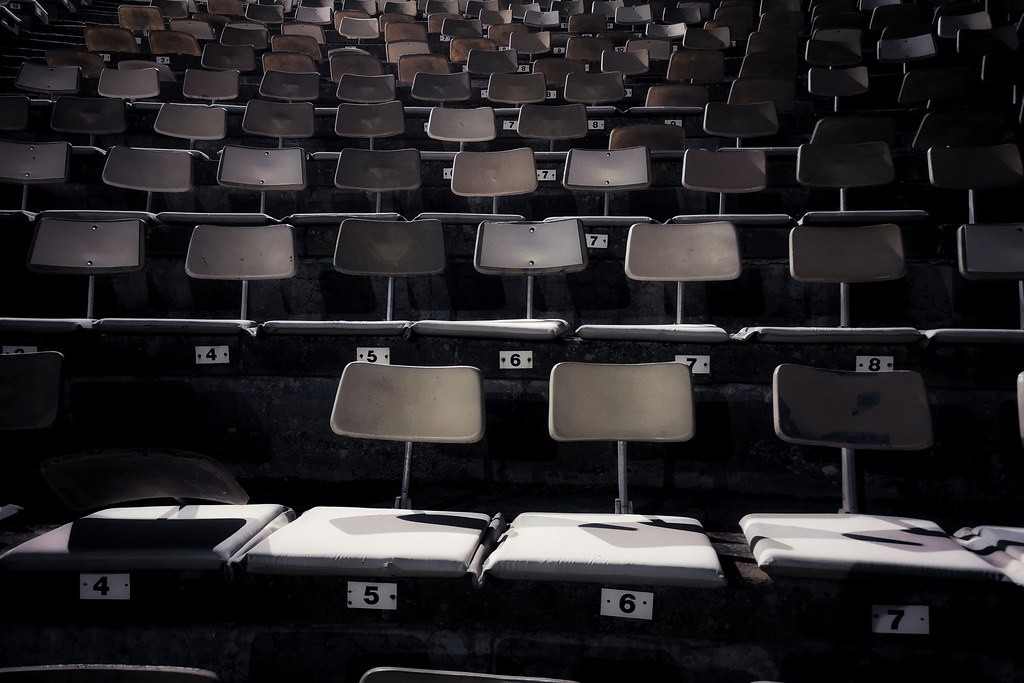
[0,0,1024,683]
